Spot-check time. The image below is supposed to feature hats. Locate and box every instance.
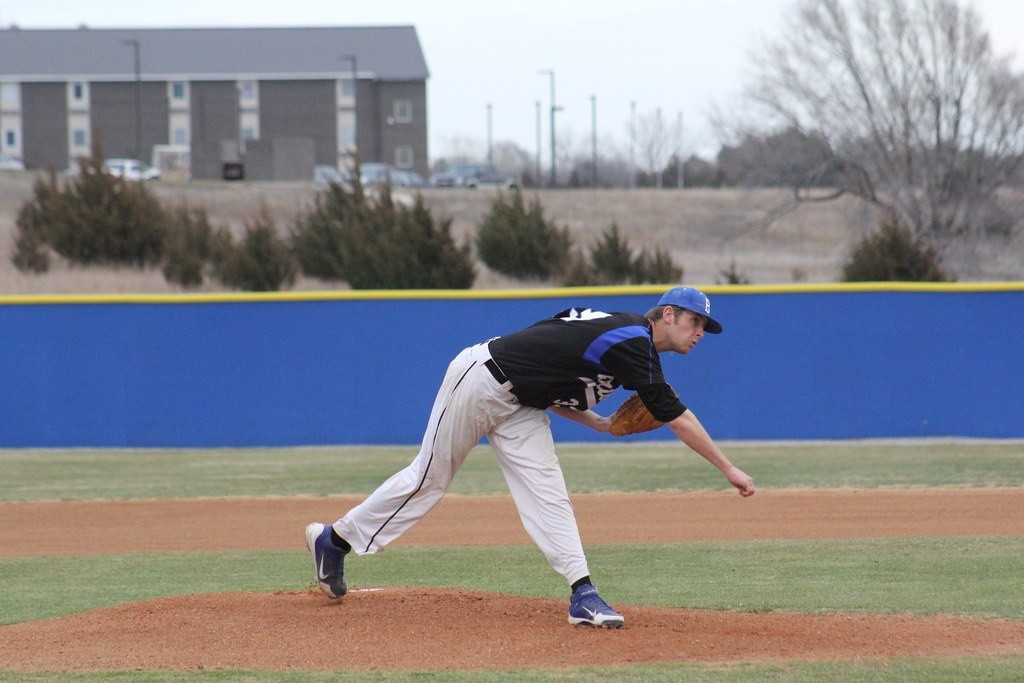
[656,286,723,334]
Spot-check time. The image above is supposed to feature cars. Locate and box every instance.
[360,161,422,187]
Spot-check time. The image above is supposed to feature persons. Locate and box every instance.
[304,287,754,629]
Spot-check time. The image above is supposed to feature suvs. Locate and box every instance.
[102,158,159,181]
[430,164,495,188]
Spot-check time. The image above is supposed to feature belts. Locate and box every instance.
[480,341,517,395]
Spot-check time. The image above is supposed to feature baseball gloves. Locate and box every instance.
[608,382,676,438]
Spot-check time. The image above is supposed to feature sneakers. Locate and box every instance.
[567,584,625,629]
[304,521,353,599]
